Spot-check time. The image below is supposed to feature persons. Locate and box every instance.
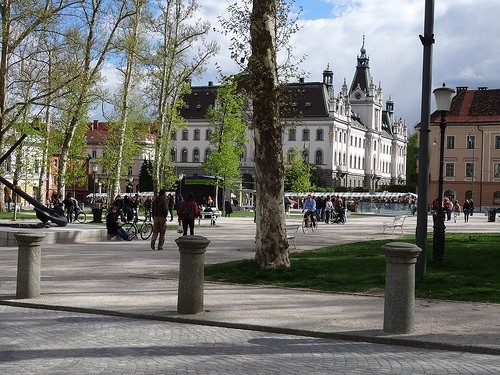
[285,194,474,228]
[57,190,239,250]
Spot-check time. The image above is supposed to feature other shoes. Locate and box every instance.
[151,241,155,250]
[129,235,135,238]
[158,246,163,250]
[127,236,133,241]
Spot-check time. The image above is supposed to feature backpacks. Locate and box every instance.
[182,201,194,220]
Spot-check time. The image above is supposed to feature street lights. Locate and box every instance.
[91,163,98,213]
[432,81,456,266]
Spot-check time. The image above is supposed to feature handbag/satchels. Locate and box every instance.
[177,225,183,233]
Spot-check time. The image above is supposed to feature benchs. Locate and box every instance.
[382,215,407,235]
[286,224,300,250]
[198,207,222,225]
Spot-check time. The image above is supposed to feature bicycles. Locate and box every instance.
[301,212,318,234]
[65,207,87,224]
[119,215,153,241]
